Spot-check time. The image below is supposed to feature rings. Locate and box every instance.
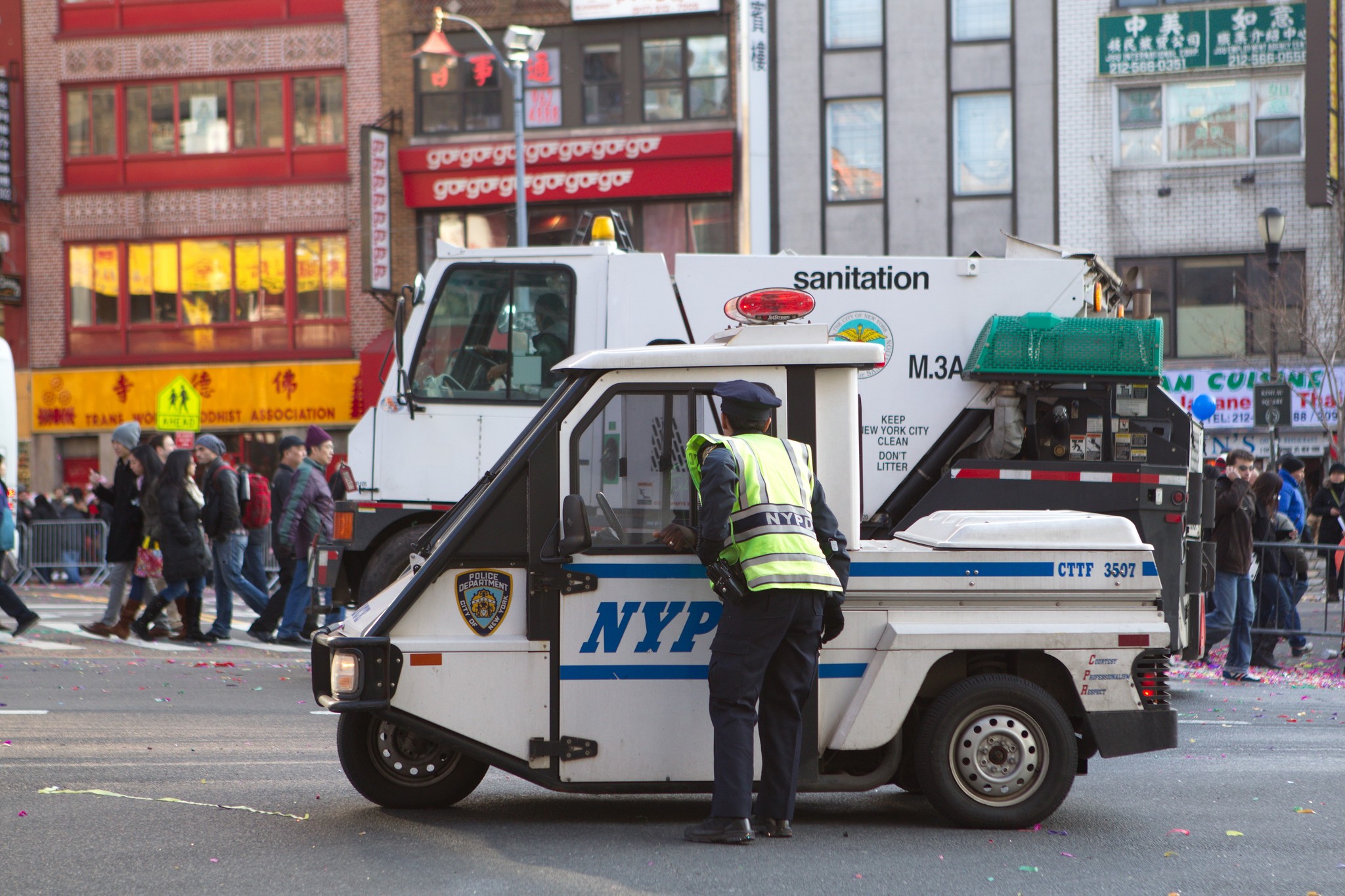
[669,541,676,546]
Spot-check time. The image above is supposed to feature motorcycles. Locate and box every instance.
[310,320,1179,831]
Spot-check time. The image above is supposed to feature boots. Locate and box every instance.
[1327,577,1339,601]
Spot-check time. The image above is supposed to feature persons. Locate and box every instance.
[0,421,346,647]
[472,293,569,389]
[1194,449,1313,682]
[647,510,698,552]
[1311,463,1345,603]
[684,380,852,845]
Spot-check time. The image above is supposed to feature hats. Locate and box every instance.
[713,380,782,421]
[278,436,306,452]
[1329,463,1345,476]
[305,425,333,449]
[195,433,226,457]
[111,421,141,451]
[1281,455,1305,474]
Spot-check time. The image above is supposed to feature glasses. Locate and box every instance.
[1238,465,1254,470]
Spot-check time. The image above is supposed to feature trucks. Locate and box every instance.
[330,206,1166,604]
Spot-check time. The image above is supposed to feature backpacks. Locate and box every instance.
[212,466,272,528]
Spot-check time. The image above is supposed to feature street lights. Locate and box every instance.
[412,4,528,249]
[1257,208,1286,460]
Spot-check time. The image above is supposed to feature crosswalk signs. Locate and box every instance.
[151,376,201,435]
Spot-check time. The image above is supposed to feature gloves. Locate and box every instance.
[821,609,844,644]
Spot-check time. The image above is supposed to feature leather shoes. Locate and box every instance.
[684,816,755,843]
[751,814,792,836]
[13,568,321,645]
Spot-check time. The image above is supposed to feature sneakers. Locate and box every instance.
[1199,642,1314,682]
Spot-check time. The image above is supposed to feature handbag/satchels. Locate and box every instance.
[1248,550,1261,582]
[133,535,164,579]
